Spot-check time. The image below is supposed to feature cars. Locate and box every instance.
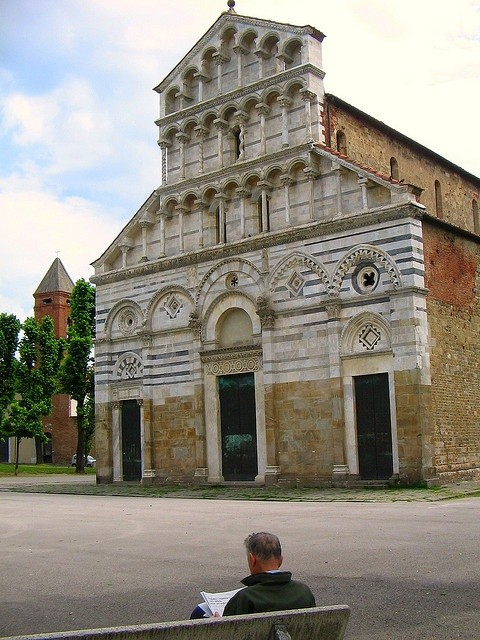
[72,453,96,469]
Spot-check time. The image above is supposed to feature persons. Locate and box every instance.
[189,530,316,620]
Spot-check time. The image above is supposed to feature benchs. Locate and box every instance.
[0,605,349,640]
